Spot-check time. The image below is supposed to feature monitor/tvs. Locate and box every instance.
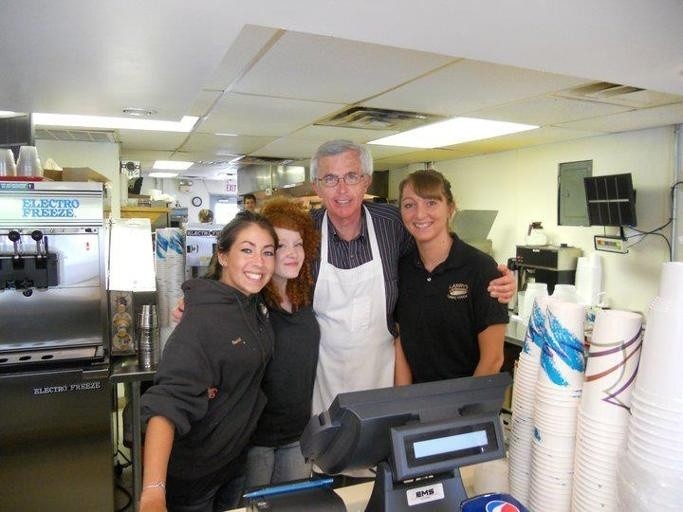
[300,372,515,478]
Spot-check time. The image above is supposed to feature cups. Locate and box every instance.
[124,198,138,207]
[155,228,185,361]
[0,146,44,178]
[510,252,683,512]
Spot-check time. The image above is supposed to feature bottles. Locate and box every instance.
[136,302,159,372]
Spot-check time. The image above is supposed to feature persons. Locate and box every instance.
[173,194,320,489]
[310,140,516,417]
[137,210,279,511]
[112,303,133,337]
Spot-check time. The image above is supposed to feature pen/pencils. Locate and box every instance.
[242,477,334,497]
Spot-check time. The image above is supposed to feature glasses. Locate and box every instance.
[315,172,364,187]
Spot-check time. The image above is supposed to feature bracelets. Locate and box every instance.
[144,481,165,488]
